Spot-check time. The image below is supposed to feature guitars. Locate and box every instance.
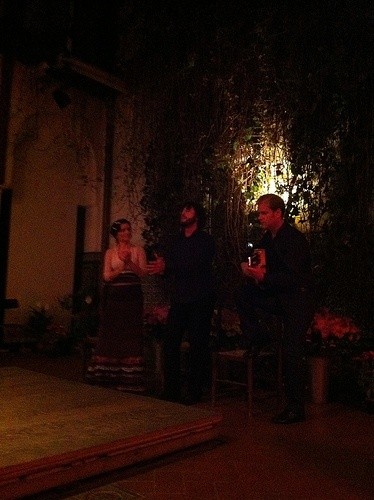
[247,248,268,275]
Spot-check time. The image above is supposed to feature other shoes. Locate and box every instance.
[114,383,145,391]
[272,404,306,423]
[159,390,180,402]
[241,336,265,359]
[180,394,199,405]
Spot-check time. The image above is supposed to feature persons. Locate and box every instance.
[88,218,146,392]
[233,193,318,425]
[146,200,217,406]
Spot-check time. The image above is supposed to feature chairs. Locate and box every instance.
[80,304,98,377]
[211,313,284,417]
